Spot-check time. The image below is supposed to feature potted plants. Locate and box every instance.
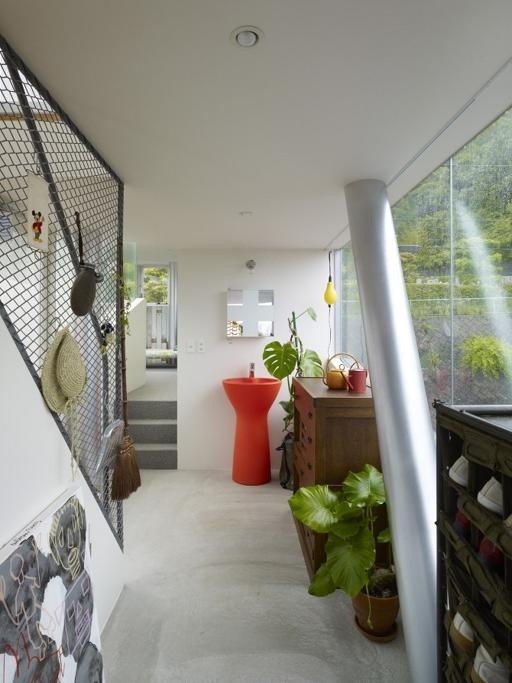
[287,464,400,643]
[263,304,324,490]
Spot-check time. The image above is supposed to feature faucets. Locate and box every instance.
[249,363,255,377]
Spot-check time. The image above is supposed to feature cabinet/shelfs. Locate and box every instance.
[291,375,387,582]
[431,399,511,682]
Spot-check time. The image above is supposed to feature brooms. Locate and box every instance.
[111,281,142,499]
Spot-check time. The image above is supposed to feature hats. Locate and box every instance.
[41,329,85,417]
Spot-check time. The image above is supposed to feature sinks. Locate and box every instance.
[222,377,282,411]
